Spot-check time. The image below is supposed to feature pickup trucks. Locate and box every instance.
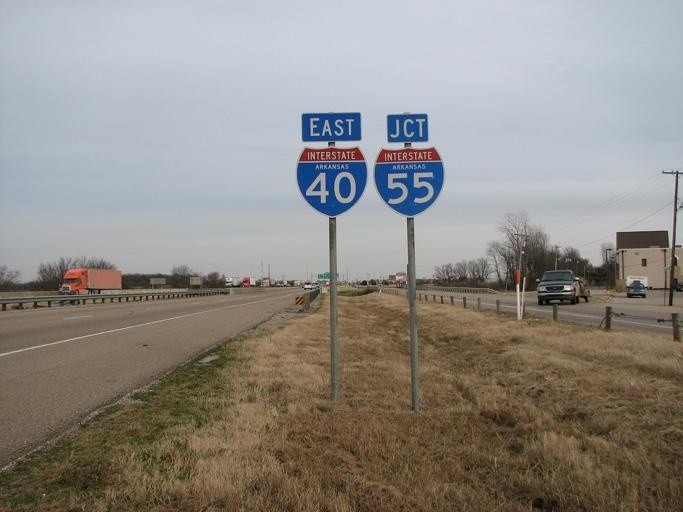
[535,269,580,305]
[626,280,647,299]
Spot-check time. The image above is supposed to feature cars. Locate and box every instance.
[301,281,324,291]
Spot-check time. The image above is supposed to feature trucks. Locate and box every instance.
[58,268,123,296]
[225,276,257,288]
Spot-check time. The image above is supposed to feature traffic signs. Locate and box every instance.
[373,147,443,219]
[301,113,363,142]
[297,147,368,218]
[387,113,429,143]
